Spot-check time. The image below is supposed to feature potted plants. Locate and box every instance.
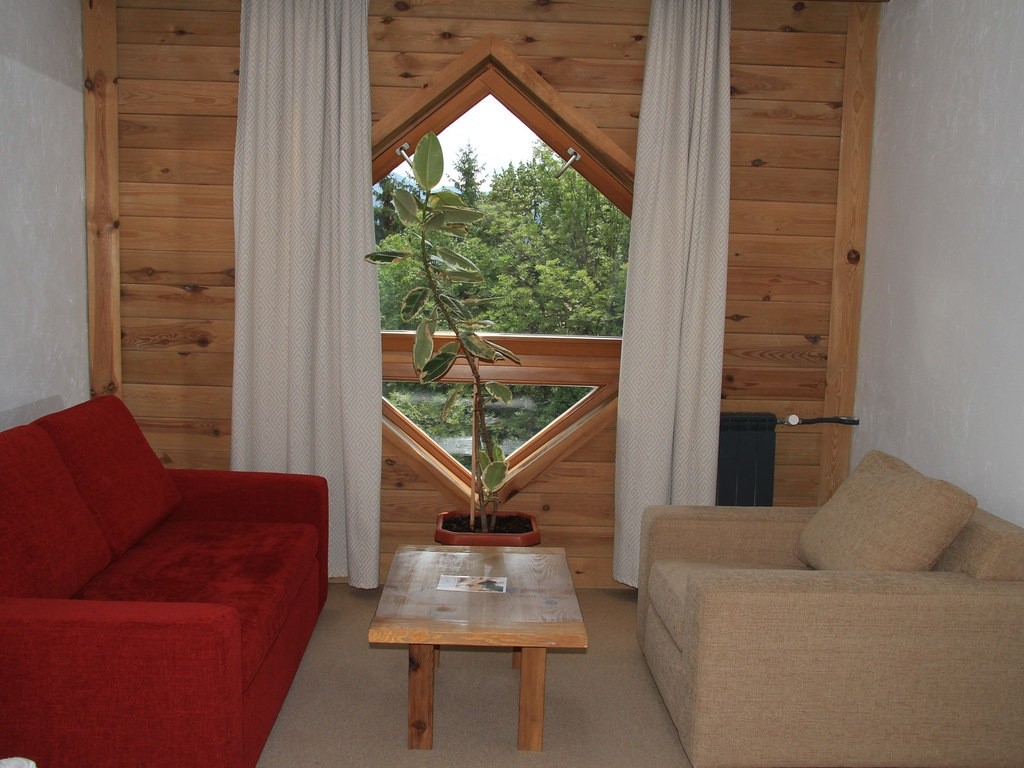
[362,130,542,547]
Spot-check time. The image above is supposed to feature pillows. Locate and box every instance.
[796,451,980,574]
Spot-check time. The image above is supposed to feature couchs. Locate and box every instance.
[639,503,1024,768]
[0,391,328,768]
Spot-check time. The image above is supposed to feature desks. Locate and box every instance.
[367,544,590,753]
